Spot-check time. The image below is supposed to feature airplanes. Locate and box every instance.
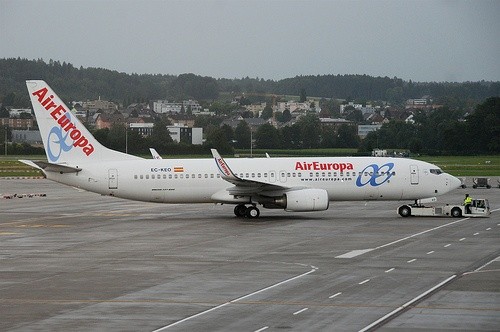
[17,78,463,222]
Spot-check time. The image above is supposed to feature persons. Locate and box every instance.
[462,193,472,213]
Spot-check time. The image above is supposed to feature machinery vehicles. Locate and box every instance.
[411,198,491,218]
